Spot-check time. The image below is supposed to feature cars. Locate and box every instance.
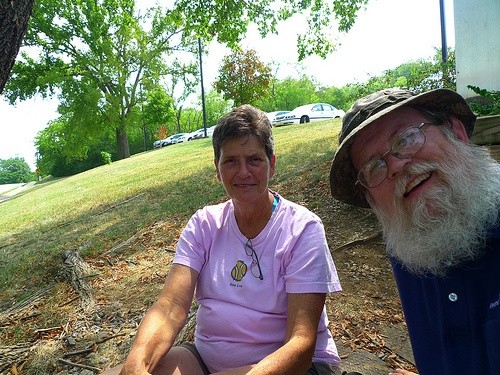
[152,125,217,149]
[267,111,292,127]
[272,103,346,128]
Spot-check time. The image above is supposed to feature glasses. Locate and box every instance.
[355,122,434,188]
[244,238,264,280]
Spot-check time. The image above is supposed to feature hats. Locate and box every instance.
[329,87,477,208]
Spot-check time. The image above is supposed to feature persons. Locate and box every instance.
[330,88,500,375]
[100,104,343,375]
